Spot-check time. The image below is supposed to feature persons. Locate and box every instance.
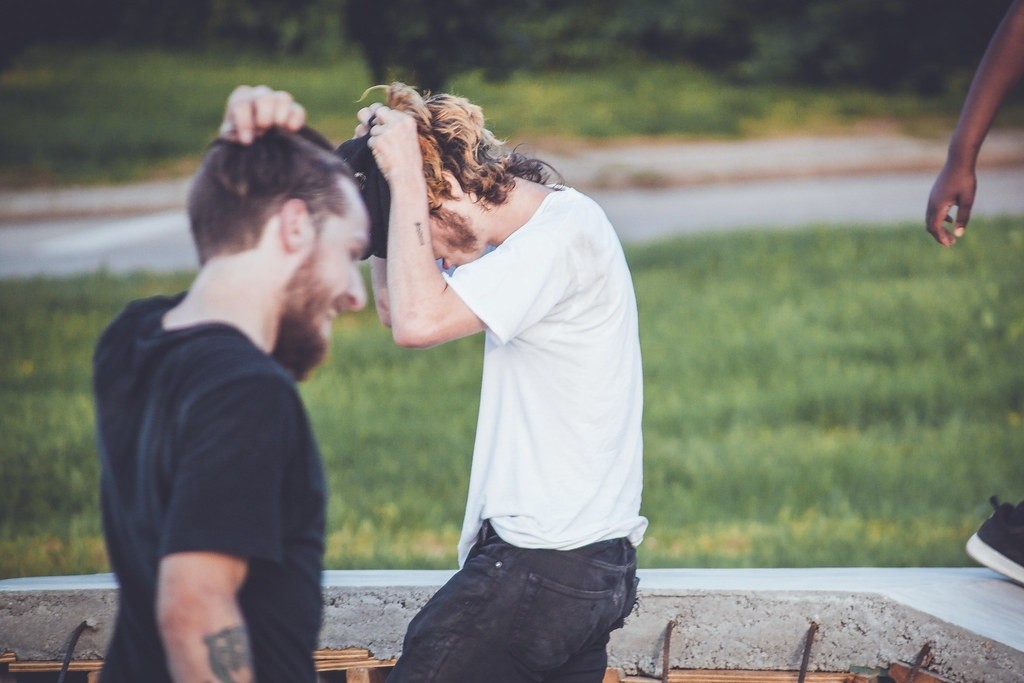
[335,81,649,683]
[90,84,366,683]
[925,0,1024,585]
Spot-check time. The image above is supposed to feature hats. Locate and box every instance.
[335,133,389,262]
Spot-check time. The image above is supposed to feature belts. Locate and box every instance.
[479,519,501,544]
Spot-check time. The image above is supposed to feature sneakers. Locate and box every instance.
[966,499,1024,586]
